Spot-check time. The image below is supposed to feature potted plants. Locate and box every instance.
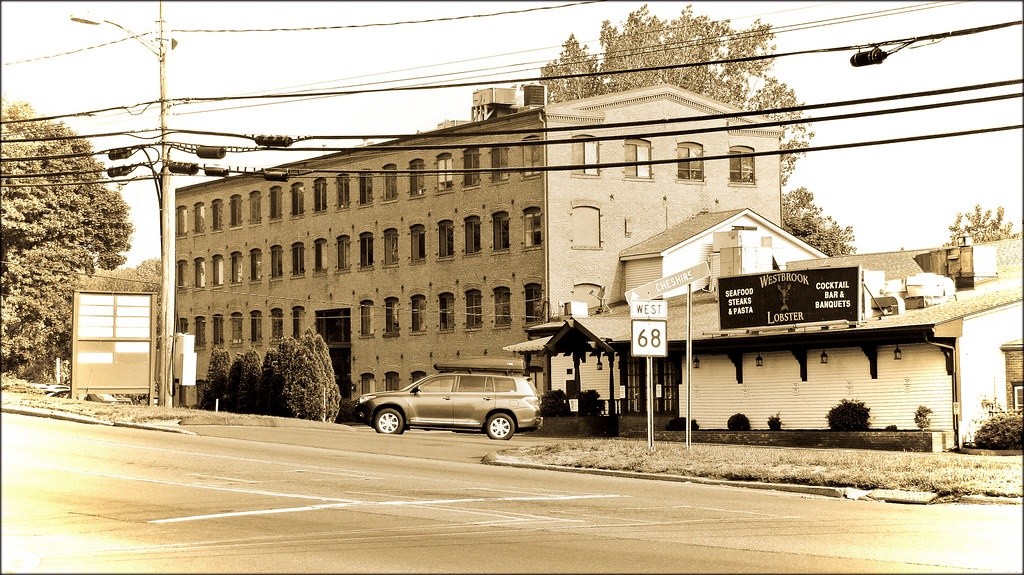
[768,412,785,430]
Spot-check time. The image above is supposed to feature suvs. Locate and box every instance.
[353,358,544,442]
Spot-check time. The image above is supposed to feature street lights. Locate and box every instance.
[70,10,177,410]
